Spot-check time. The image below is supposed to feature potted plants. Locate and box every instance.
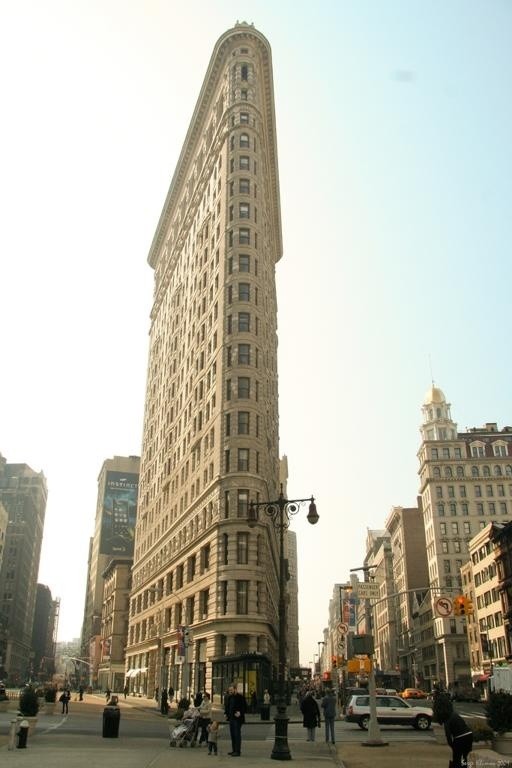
[41,686,58,716]
[14,683,41,735]
[1,694,11,713]
[429,693,455,746]
[478,687,512,757]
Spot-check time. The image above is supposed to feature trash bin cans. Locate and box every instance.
[46,688,57,702]
[103,705,120,738]
[259,704,270,720]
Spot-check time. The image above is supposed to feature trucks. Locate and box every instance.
[486,657,512,702]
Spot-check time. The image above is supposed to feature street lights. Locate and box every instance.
[340,583,354,686]
[308,640,325,670]
[146,585,191,701]
[246,480,324,761]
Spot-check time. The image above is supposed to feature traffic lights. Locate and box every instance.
[332,655,337,668]
[465,598,475,613]
[452,597,459,616]
[458,596,466,615]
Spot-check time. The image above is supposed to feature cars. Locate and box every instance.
[330,682,484,701]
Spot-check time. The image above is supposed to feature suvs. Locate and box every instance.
[343,691,435,732]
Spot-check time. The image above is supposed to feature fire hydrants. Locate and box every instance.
[14,718,30,748]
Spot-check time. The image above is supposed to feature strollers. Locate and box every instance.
[166,705,202,747]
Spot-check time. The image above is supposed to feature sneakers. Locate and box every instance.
[231,751,241,757]
[228,751,234,755]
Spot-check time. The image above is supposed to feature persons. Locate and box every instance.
[206,720,219,755]
[123,686,128,699]
[440,709,474,768]
[104,688,112,701]
[77,686,84,701]
[224,686,247,756]
[194,693,206,741]
[161,690,168,714]
[300,691,320,741]
[321,690,337,744]
[169,687,174,703]
[195,692,212,747]
[62,688,71,714]
